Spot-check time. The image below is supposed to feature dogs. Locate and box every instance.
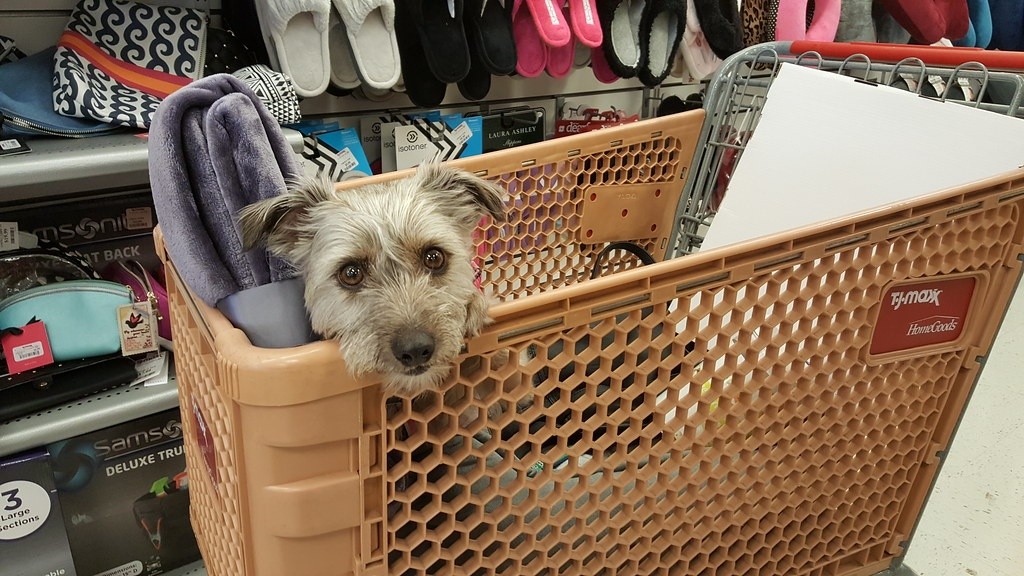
[236,158,536,440]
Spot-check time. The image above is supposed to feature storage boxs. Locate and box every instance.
[43,407,201,576]
[0,443,77,576]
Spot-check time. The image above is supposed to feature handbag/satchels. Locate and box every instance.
[499,240,698,459]
[0,1,301,361]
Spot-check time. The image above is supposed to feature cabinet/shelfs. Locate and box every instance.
[0,0,303,463]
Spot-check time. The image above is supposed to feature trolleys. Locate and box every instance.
[152,35,1023,576]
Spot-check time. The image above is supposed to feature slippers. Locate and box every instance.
[253,0,743,106]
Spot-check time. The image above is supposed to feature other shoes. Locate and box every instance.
[658,91,759,212]
